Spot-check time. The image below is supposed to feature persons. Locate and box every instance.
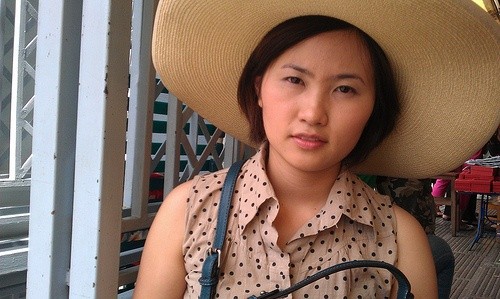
[374,135,500,298]
[132,15,438,299]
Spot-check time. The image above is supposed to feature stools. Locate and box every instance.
[430,172,460,237]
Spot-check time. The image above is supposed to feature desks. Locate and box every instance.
[456,191,500,251]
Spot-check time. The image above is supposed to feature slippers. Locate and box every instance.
[436,212,479,226]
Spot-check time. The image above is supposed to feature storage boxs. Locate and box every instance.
[454,166,500,192]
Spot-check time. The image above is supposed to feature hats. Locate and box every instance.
[151,0,500,180]
